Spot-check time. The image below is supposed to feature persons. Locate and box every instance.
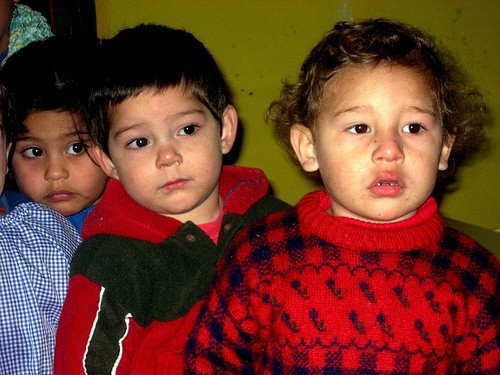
[181,16,500,375]
[0,0,109,375]
[53,20,293,375]
